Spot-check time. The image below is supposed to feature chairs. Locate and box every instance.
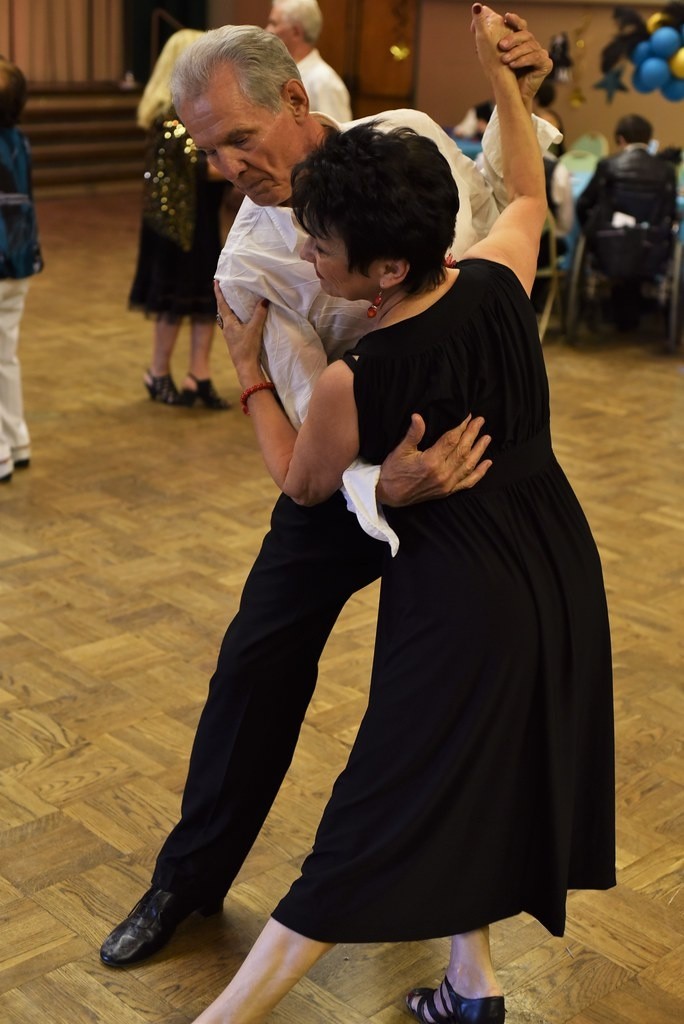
[524,208,563,346]
[567,227,681,355]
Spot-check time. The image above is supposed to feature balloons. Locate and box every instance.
[630,27,684,102]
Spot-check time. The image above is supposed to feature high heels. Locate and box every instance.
[182,372,232,410]
[407,980,506,1024]
[143,369,183,405]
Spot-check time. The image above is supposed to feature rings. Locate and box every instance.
[217,312,222,327]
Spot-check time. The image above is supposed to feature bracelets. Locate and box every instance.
[239,380,275,415]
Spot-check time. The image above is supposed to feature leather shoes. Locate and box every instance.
[100,885,223,969]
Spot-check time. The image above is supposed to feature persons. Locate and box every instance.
[127,29,234,411]
[264,0,352,123]
[573,114,677,334]
[0,53,44,484]
[453,61,570,315]
[188,3,618,1023]
[96,11,554,972]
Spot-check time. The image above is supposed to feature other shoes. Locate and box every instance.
[1,461,13,483]
[12,448,32,466]
